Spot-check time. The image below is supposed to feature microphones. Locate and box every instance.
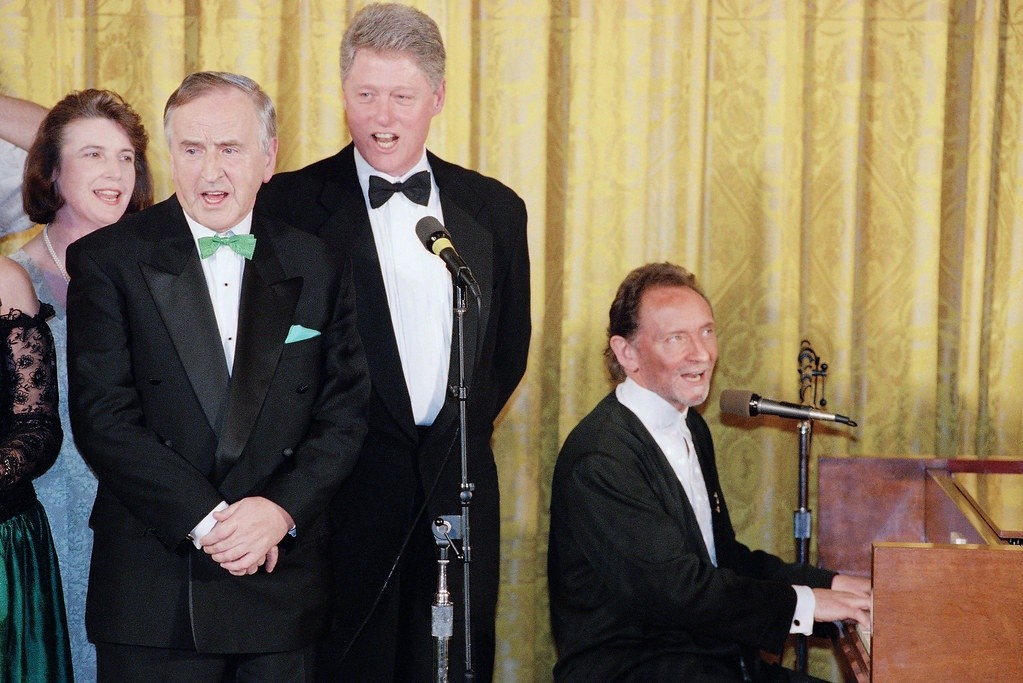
[719,388,857,427]
[415,216,483,300]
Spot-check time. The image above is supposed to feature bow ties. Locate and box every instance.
[198,234,257,260]
[368,170,431,209]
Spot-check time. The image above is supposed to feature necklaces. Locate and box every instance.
[43,223,71,285]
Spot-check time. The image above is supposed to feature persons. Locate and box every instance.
[0,95,50,247]
[264,3,532,683]
[0,254,75,683]
[65,72,371,683]
[9,88,155,683]
[545,261,872,683]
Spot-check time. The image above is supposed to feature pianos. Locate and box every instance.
[815,453,1023,683]
